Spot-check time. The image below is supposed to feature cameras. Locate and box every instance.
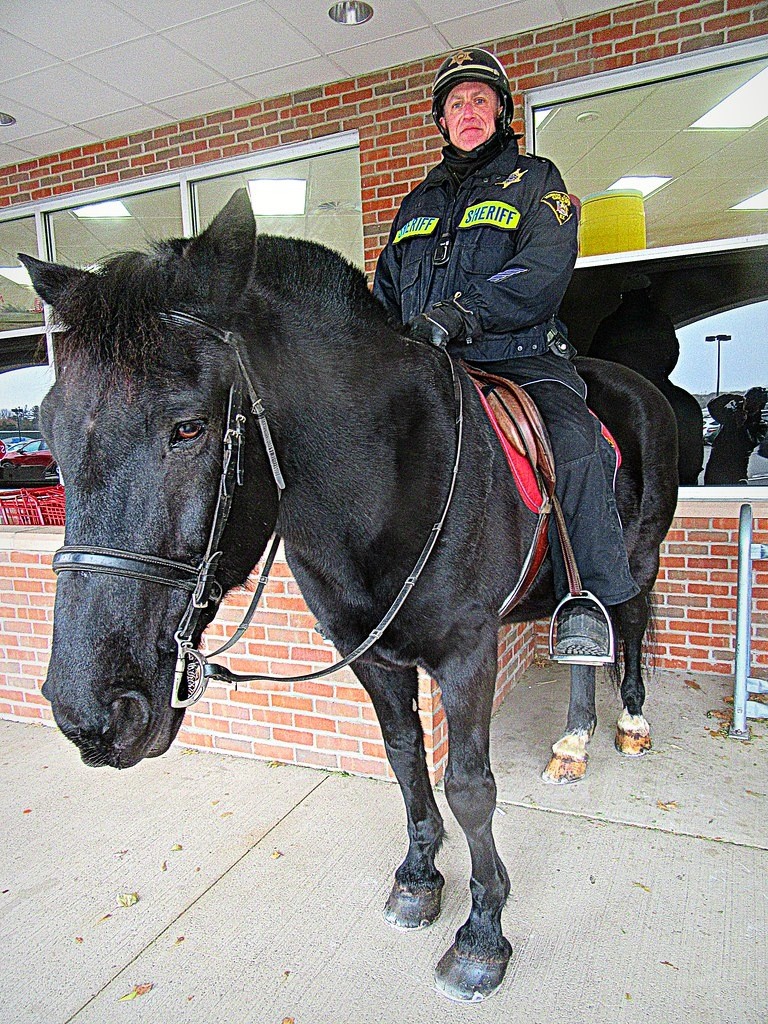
[737,403,744,415]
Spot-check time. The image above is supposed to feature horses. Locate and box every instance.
[14,185,680,1005]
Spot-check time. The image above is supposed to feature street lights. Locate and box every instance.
[704,334,732,397]
[11,408,23,442]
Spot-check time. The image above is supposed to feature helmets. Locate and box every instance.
[620,273,652,292]
[432,48,514,128]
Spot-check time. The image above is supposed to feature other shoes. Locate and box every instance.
[556,617,608,656]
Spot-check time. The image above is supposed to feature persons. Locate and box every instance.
[373,48,642,656]
[705,387,768,487]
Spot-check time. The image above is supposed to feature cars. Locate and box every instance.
[701,406,721,445]
[0,436,59,476]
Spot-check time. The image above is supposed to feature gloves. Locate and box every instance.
[399,306,463,348]
